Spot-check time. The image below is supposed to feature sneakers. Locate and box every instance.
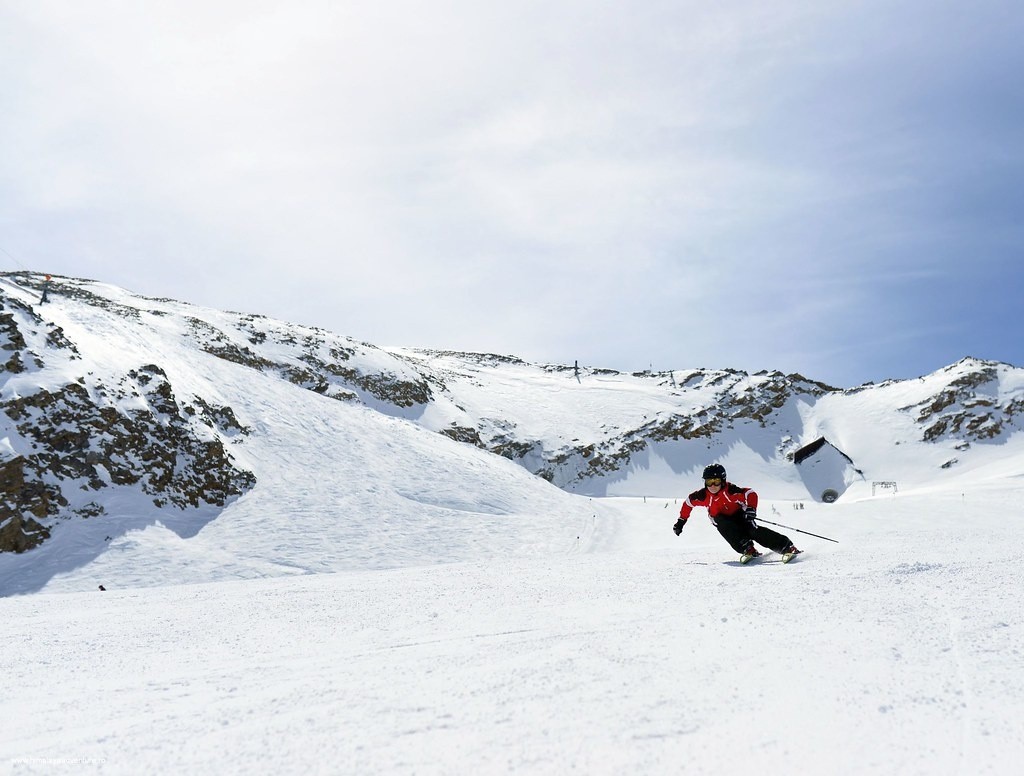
[788,546,797,554]
[746,548,757,555]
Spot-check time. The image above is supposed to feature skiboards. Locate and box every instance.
[740,547,800,566]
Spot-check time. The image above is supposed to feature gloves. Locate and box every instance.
[745,507,756,521]
[672,517,687,536]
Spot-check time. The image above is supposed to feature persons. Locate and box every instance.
[674,464,799,555]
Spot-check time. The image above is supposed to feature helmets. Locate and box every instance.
[701,463,726,479]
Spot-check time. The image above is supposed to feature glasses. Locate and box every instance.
[705,478,721,487]
